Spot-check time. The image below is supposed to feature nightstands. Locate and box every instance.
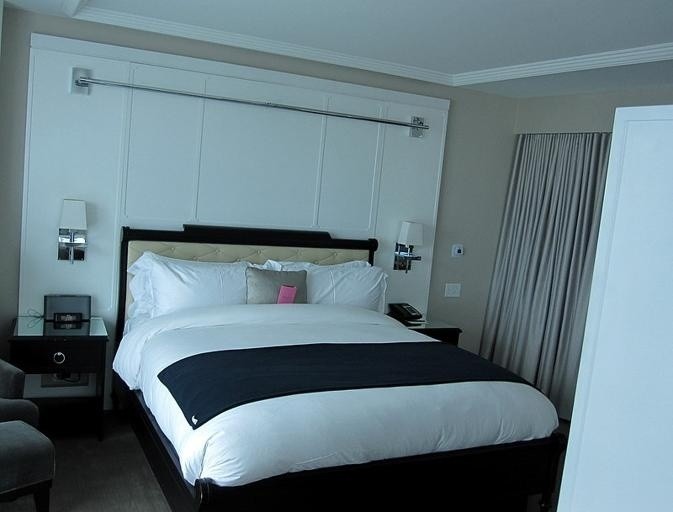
[10,316,108,443]
[408,322,462,348]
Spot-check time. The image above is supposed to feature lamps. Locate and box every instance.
[394,221,424,274]
[60,198,88,264]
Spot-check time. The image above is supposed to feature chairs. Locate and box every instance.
[0,360,56,511]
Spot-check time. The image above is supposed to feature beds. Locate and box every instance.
[112,223,559,511]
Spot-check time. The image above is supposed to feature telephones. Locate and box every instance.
[388,302,422,320]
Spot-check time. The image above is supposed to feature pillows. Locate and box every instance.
[126,249,391,331]
